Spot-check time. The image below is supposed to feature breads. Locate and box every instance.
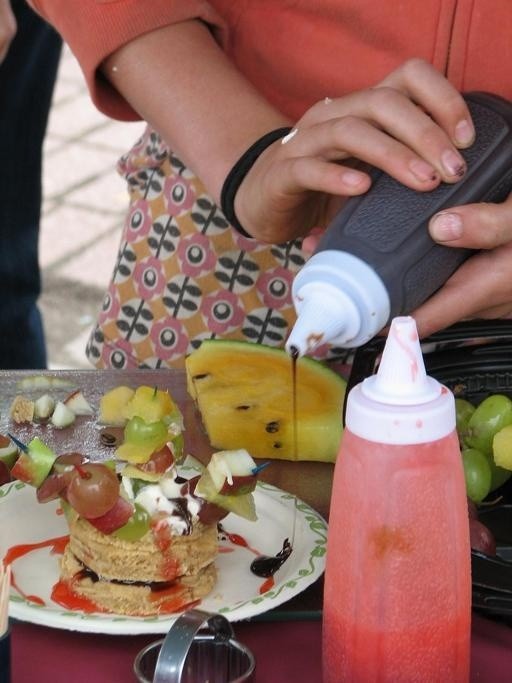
[10,385,258,616]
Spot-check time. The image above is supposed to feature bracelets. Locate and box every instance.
[220,124,293,237]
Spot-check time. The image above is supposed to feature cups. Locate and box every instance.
[132,608,254,683]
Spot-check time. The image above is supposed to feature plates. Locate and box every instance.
[0,462,328,637]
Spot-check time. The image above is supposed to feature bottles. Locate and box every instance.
[284,89,511,361]
[323,316,472,683]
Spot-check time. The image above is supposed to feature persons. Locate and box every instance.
[0,0,64,368]
[29,0,512,371]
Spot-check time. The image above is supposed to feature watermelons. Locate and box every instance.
[185,340,348,463]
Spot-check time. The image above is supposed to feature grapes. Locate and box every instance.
[450,394,512,503]
